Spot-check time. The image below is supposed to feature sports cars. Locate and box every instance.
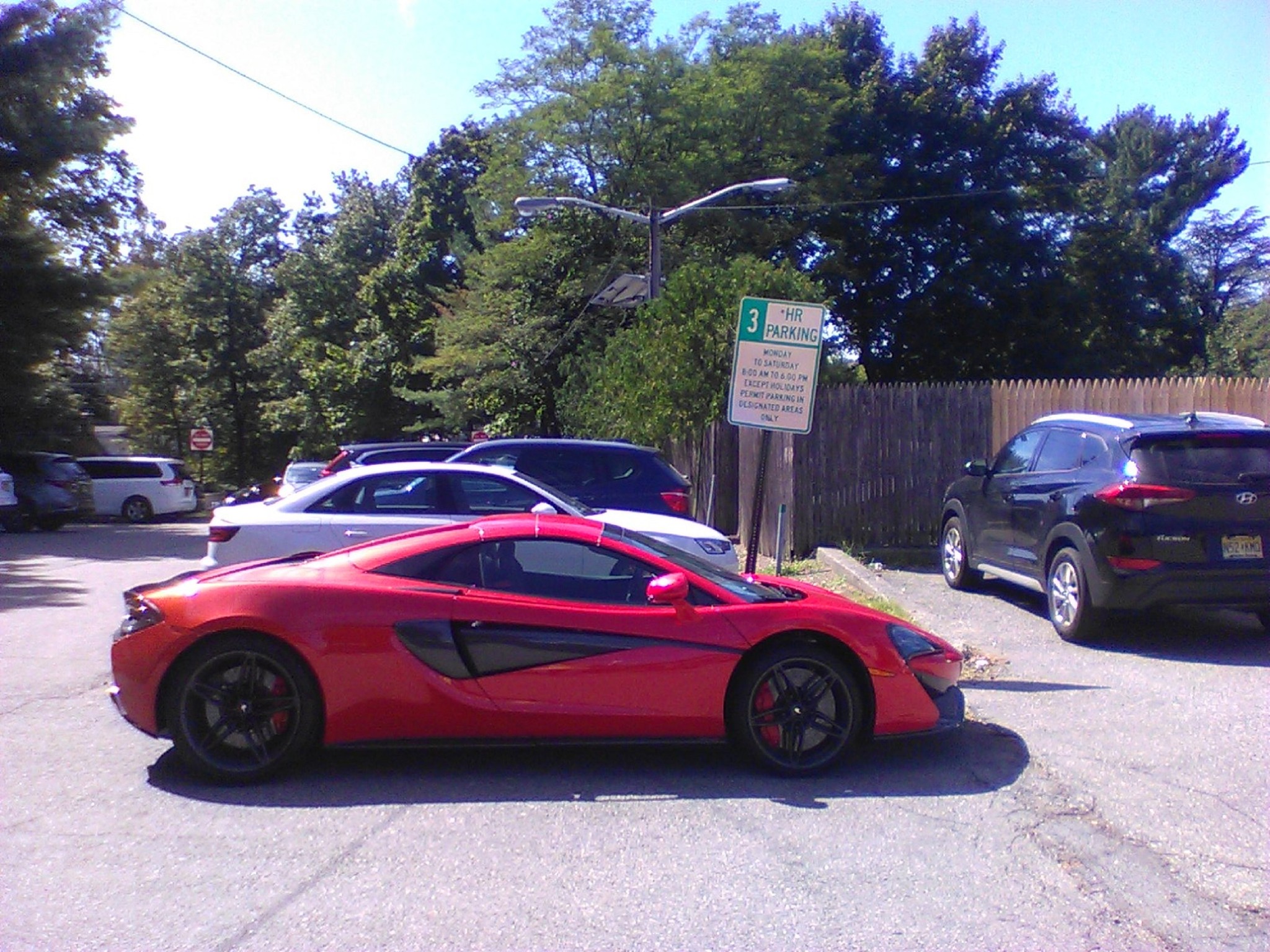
[106,510,964,777]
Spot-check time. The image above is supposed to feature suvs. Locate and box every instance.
[0,451,97,533]
[938,409,1270,642]
[316,443,473,484]
[369,437,695,517]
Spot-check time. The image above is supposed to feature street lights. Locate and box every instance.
[512,178,814,303]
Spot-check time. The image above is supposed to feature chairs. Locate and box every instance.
[499,542,532,592]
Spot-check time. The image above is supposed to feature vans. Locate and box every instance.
[76,457,197,524]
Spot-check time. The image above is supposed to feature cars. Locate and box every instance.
[201,461,739,584]
[220,460,330,506]
[0,467,19,506]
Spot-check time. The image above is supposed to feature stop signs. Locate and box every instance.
[190,429,214,451]
[471,432,490,444]
